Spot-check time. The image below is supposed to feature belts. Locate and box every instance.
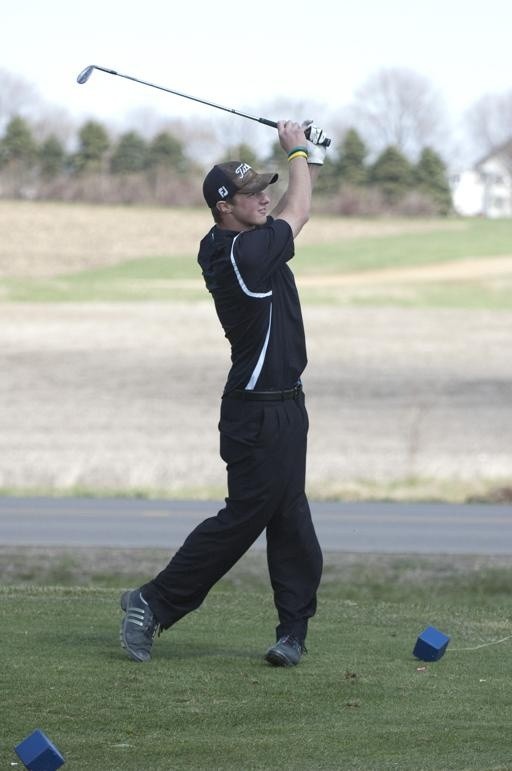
[227,384,302,402]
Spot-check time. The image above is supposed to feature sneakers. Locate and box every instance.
[263,632,308,667]
[120,587,163,663]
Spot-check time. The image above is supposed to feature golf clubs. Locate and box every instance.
[78,64,330,149]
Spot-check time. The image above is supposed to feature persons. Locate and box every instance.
[121,120,323,669]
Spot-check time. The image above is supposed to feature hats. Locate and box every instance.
[203,162,278,208]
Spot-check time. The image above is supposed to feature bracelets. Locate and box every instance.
[288,151,307,162]
[287,146,308,157]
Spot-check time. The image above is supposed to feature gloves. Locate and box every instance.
[300,119,326,166]
